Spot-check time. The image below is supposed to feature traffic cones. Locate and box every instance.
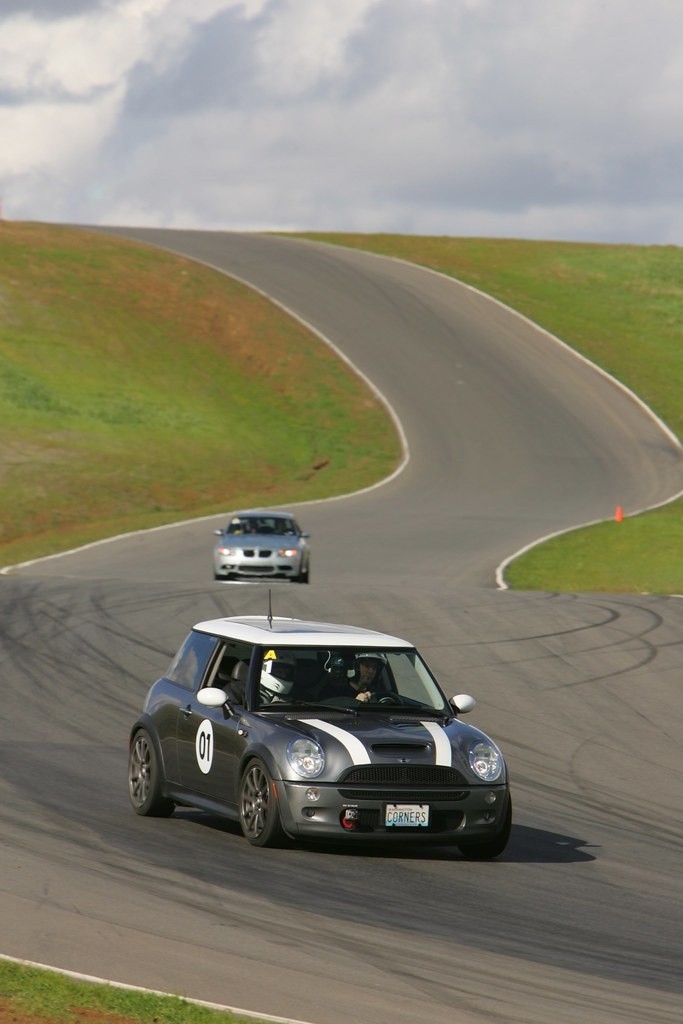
[615,505,623,522]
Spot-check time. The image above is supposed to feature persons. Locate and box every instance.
[249,522,259,533]
[272,521,290,535]
[222,650,299,706]
[318,653,386,704]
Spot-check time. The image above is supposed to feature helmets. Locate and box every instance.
[259,650,296,695]
[347,652,387,684]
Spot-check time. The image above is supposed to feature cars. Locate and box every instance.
[212,512,312,584]
[127,615,512,858]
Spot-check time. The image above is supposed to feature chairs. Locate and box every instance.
[217,656,316,707]
[259,526,273,532]
